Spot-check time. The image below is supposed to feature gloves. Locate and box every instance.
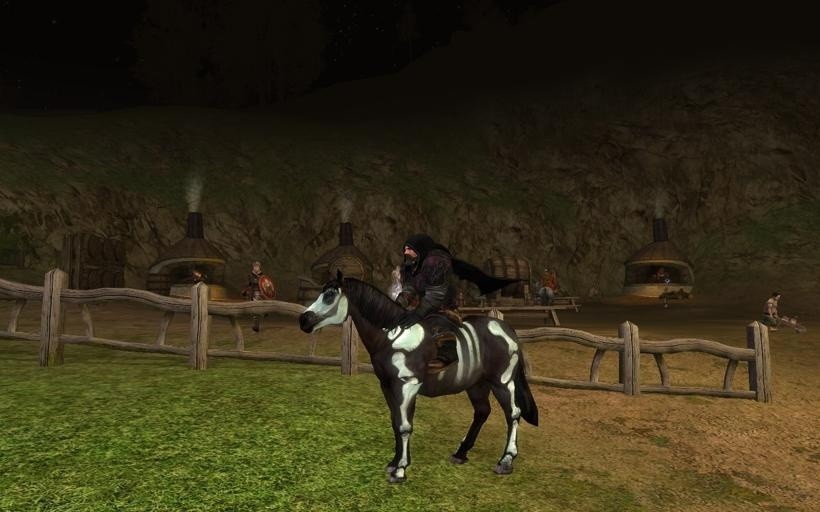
[398,311,422,329]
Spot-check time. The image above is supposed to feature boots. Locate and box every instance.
[427,335,461,375]
[251,319,259,332]
